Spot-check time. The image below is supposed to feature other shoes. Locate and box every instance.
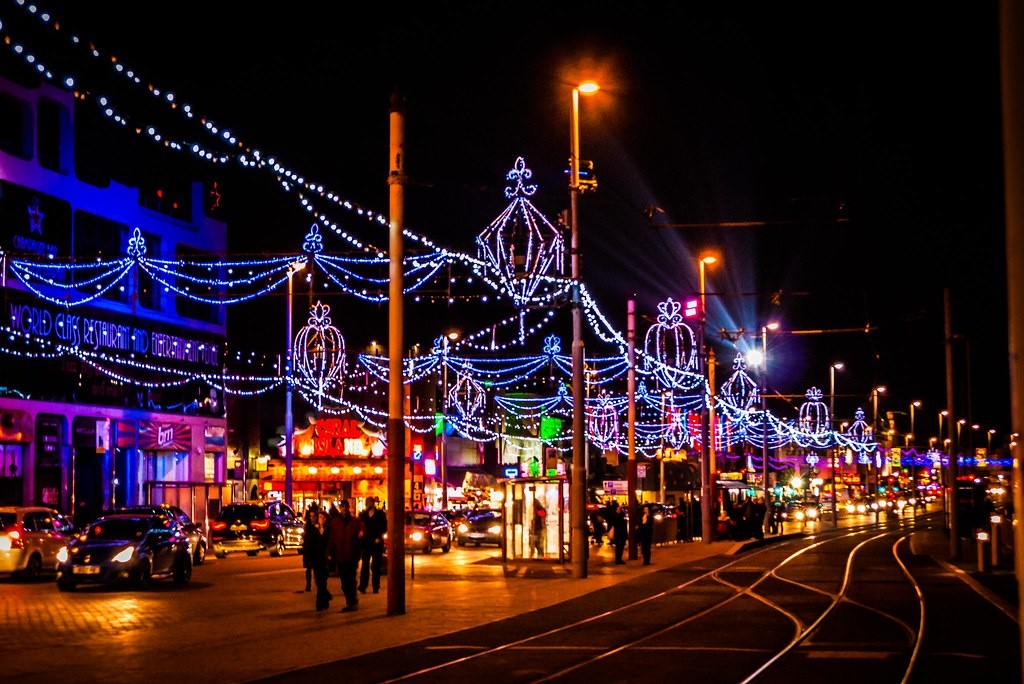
[342,607,359,612]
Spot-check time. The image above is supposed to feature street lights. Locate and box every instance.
[761,321,779,499]
[830,361,844,525]
[570,80,604,579]
[286,261,308,507]
[699,257,716,544]
[871,386,1021,524]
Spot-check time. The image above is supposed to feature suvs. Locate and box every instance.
[209,501,305,560]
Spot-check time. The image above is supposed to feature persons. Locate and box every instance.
[534,499,547,559]
[303,496,388,612]
[742,497,785,539]
[591,501,652,567]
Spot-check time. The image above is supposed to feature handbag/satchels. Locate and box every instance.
[634,532,640,544]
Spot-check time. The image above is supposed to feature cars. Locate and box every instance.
[120,504,210,565]
[776,490,939,523]
[457,508,502,550]
[53,513,194,592]
[381,509,453,555]
[0,507,79,581]
[582,502,680,543]
